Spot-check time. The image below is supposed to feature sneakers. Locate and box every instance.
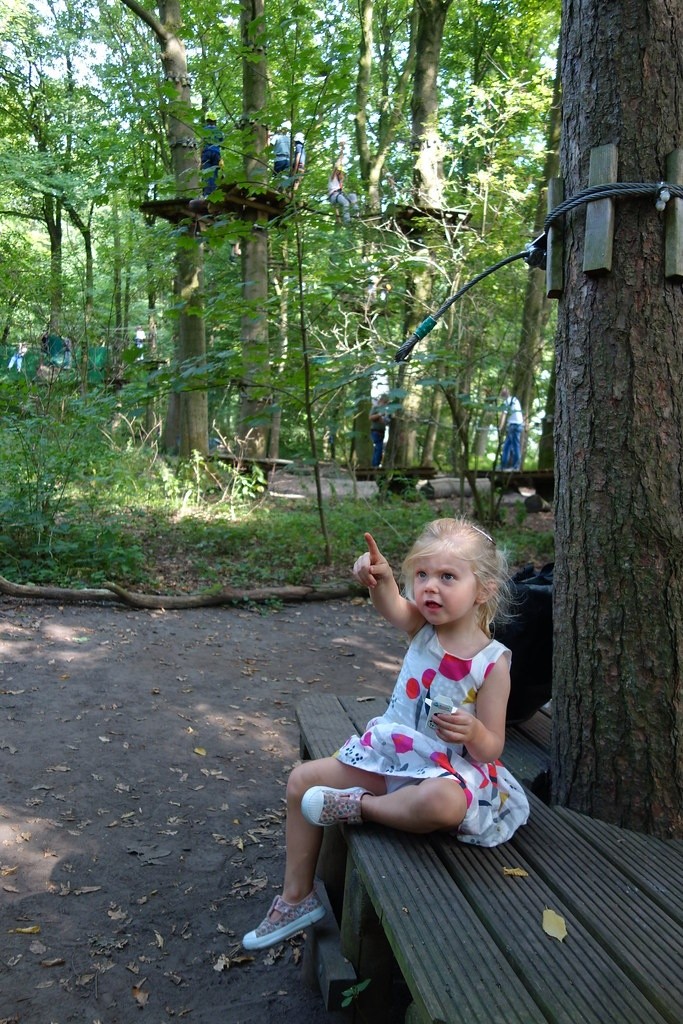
[242,889,326,950]
[301,787,375,828]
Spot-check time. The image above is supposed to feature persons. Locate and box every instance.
[7,342,27,373]
[243,519,530,949]
[289,132,305,197]
[135,326,145,361]
[498,389,523,472]
[328,150,359,225]
[368,394,388,467]
[200,111,224,199]
[266,127,290,193]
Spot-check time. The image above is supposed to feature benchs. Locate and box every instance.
[295,693,683,1024]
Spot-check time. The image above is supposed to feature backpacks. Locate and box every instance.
[490,561,553,727]
